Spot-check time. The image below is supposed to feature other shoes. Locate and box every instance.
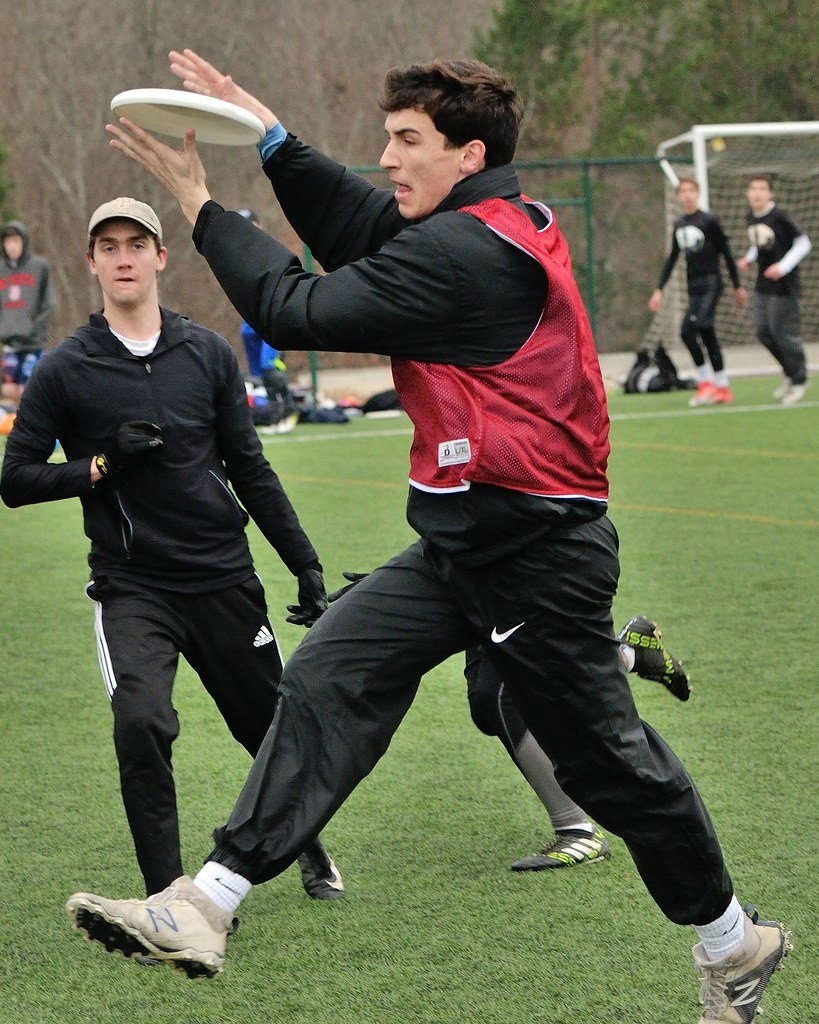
[689,382,732,407]
[772,377,809,404]
[262,409,299,434]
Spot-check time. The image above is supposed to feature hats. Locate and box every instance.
[88,197,163,247]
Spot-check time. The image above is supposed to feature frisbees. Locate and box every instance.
[107,85,268,148]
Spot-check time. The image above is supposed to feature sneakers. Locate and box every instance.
[616,617,692,702]
[693,905,794,1024]
[510,824,612,873]
[65,875,240,980]
[297,839,346,901]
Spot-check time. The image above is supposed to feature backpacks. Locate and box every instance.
[624,347,693,394]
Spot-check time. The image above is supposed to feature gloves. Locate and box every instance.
[286,569,328,628]
[96,420,164,481]
[328,571,370,603]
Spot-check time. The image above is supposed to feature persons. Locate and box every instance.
[236,209,299,433]
[737,175,812,404]
[285,573,693,871]
[648,175,748,409]
[0,221,53,414]
[64,48,794,1024]
[2,196,344,901]
